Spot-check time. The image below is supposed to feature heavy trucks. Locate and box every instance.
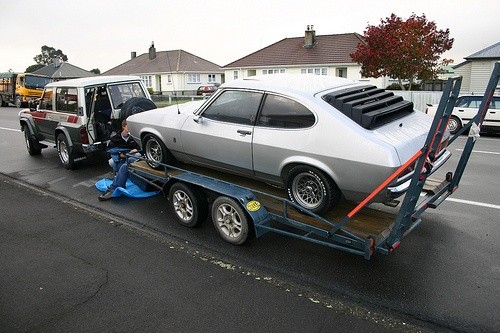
[0,73,50,108]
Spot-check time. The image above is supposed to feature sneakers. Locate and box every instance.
[99,189,115,201]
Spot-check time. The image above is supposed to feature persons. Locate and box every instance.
[98,157,139,201]
[107,119,141,175]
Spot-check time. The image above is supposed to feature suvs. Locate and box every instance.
[18,75,158,171]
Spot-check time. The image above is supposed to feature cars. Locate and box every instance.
[425,91,500,136]
[197,85,218,95]
[126,73,452,219]
